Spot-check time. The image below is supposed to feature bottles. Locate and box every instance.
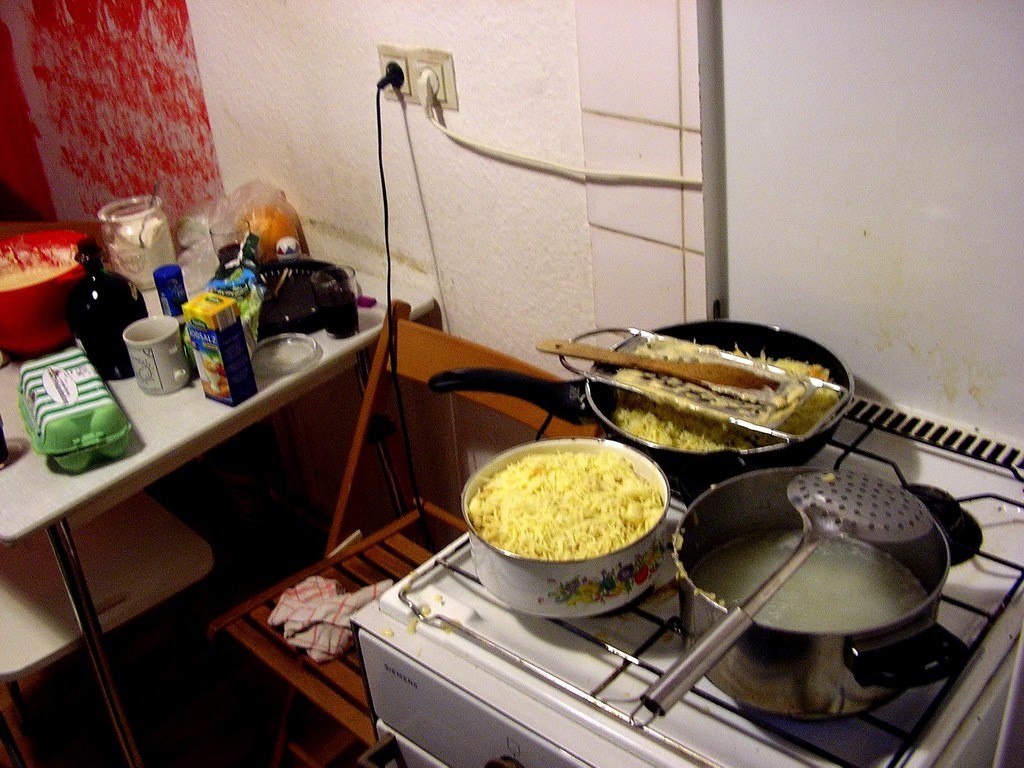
[66,236,149,381]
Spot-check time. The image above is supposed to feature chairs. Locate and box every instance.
[0,488,212,767]
[202,299,600,767]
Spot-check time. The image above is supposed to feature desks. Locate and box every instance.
[0,264,434,767]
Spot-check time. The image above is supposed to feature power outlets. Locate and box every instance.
[376,44,459,113]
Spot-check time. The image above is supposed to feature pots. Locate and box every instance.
[672,466,982,723]
[429,319,856,495]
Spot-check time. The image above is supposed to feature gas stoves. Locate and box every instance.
[346,397,1023,768]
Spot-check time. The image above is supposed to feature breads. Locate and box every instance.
[234,206,302,263]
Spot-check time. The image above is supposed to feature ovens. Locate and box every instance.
[355,719,444,768]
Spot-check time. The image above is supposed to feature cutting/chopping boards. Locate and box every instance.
[1,489,214,683]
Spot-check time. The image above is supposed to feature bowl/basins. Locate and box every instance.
[459,435,673,618]
[1,230,86,354]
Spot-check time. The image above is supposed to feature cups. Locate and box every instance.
[310,265,361,340]
[97,195,176,290]
[209,218,251,275]
[122,315,189,395]
